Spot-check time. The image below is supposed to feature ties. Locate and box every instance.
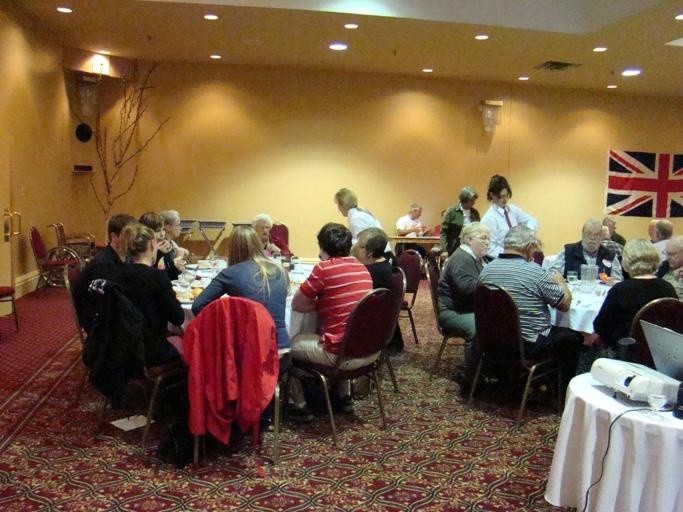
[503,205,513,228]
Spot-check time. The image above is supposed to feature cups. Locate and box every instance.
[209,255,219,278]
[567,271,578,294]
[595,286,608,300]
[182,269,196,291]
[648,379,669,420]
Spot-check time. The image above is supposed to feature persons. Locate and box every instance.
[594,238,679,362]
[192,225,291,357]
[354,227,394,289]
[71,214,142,411]
[441,185,480,260]
[161,209,191,264]
[251,213,281,259]
[334,188,405,353]
[602,217,626,245]
[427,210,446,267]
[139,212,187,276]
[548,218,624,285]
[476,225,584,400]
[657,237,683,301]
[395,203,430,276]
[281,223,374,421]
[603,226,623,257]
[480,174,540,263]
[648,218,658,244]
[116,225,185,369]
[437,221,490,393]
[654,219,674,262]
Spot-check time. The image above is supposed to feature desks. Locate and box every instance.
[544,369,683,512]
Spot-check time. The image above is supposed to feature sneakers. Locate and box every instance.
[336,394,354,413]
[291,405,315,423]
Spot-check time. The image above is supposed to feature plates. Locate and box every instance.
[174,287,200,303]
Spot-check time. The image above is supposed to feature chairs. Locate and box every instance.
[63,262,89,408]
[29,226,78,296]
[74,278,178,447]
[0,286,19,333]
[391,249,422,344]
[179,297,279,466]
[427,255,468,366]
[468,281,562,426]
[630,300,683,368]
[393,265,407,337]
[282,288,396,446]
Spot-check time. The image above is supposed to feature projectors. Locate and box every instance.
[589,358,683,404]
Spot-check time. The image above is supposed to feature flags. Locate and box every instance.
[604,149,683,219]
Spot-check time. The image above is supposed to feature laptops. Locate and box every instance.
[642,319,683,381]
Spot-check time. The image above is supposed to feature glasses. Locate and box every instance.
[171,223,181,227]
[357,243,368,250]
